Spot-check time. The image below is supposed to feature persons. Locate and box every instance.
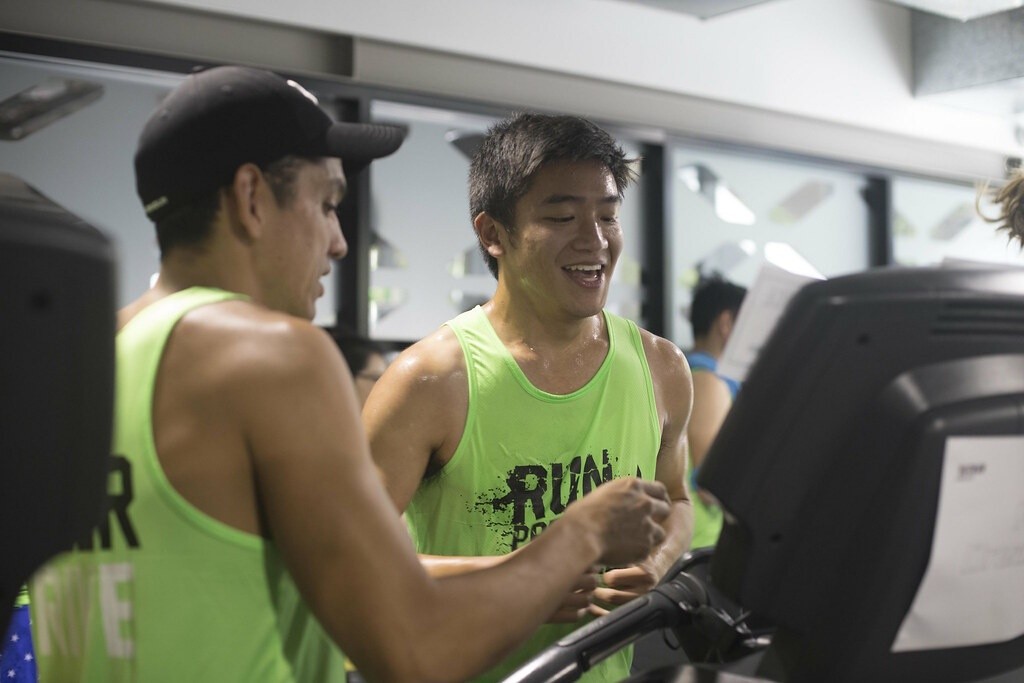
[342,109,698,683]
[23,64,675,683]
[330,338,393,683]
[665,272,752,511]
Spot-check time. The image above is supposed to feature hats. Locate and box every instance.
[134,69,407,221]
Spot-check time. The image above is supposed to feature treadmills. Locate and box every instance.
[500,260,1024,683]
